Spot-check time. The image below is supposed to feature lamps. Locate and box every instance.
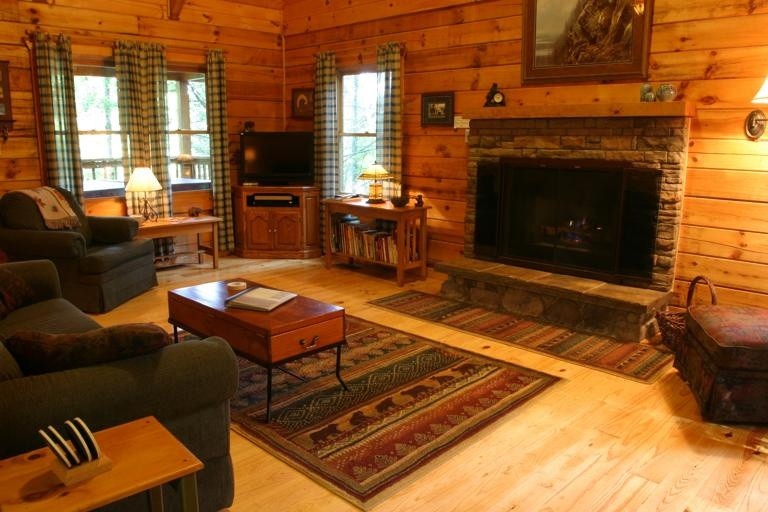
[125,167,163,223]
[358,160,396,204]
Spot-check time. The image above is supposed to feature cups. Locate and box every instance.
[130,215,143,226]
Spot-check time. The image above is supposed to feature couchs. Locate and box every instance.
[0,185,158,314]
[0,258,241,512]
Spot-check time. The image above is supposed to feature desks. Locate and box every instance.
[322,194,432,287]
[0,415,204,511]
[133,212,224,285]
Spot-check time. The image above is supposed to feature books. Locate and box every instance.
[334,216,399,264]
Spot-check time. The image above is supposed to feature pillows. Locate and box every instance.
[0,322,171,377]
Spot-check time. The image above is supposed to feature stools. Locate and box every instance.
[671,303,768,425]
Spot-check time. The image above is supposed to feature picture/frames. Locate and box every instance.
[520,1,656,88]
[290,87,315,121]
[421,91,454,128]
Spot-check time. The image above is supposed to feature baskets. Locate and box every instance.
[655,275,718,352]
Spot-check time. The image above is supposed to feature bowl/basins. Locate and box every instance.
[391,197,409,208]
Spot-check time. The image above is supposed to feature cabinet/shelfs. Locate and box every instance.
[230,185,321,259]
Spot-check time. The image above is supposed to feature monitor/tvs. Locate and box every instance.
[240,131,315,187]
